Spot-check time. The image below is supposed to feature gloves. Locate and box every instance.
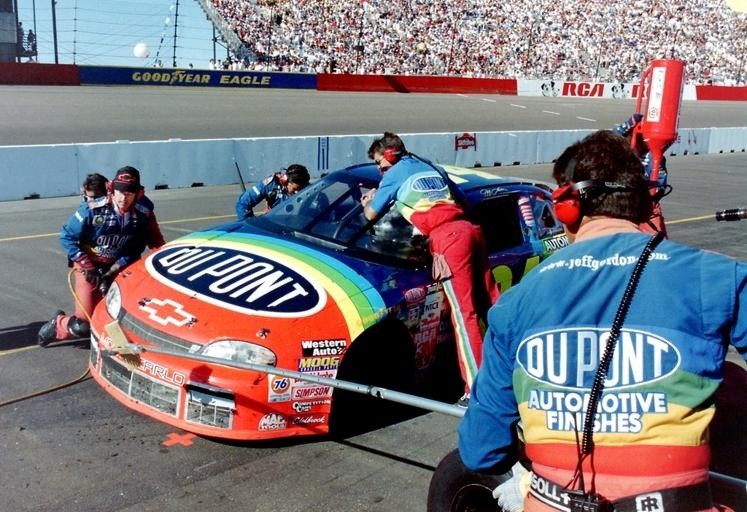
[97,263,123,296]
[75,253,102,289]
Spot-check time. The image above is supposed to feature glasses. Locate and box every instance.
[374,154,386,165]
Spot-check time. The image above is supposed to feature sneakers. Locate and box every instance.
[457,393,471,409]
[37,310,66,348]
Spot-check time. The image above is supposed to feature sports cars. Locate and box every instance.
[87,162,601,442]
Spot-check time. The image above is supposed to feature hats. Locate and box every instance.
[112,166,140,193]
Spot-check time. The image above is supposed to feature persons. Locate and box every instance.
[18,22,25,46]
[612,114,680,241]
[27,29,36,52]
[38,174,155,346]
[360,131,501,409]
[80,174,113,202]
[456,129,747,512]
[210,1,746,86]
[189,63,193,69]
[155,60,165,67]
[235,163,330,225]
[107,166,166,250]
